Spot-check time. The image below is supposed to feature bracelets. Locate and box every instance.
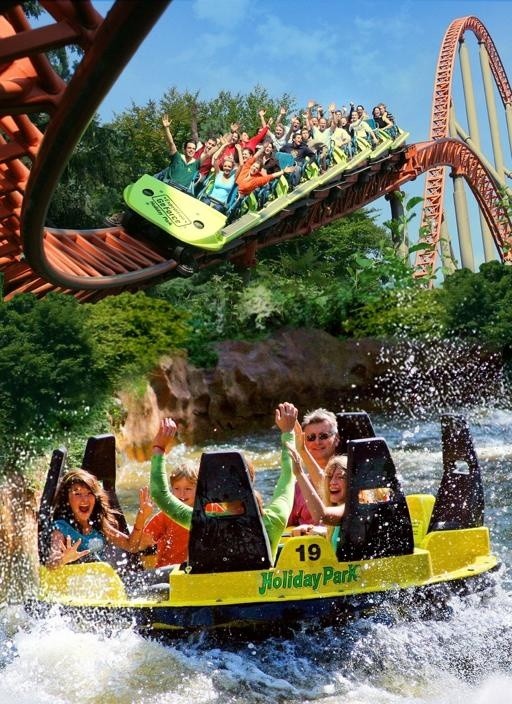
[152,445,166,453]
[134,523,144,531]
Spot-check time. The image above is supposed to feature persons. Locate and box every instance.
[150,402,297,565]
[141,462,199,570]
[44,470,153,570]
[285,408,347,553]
[162,101,396,215]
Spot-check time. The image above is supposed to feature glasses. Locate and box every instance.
[307,433,330,441]
